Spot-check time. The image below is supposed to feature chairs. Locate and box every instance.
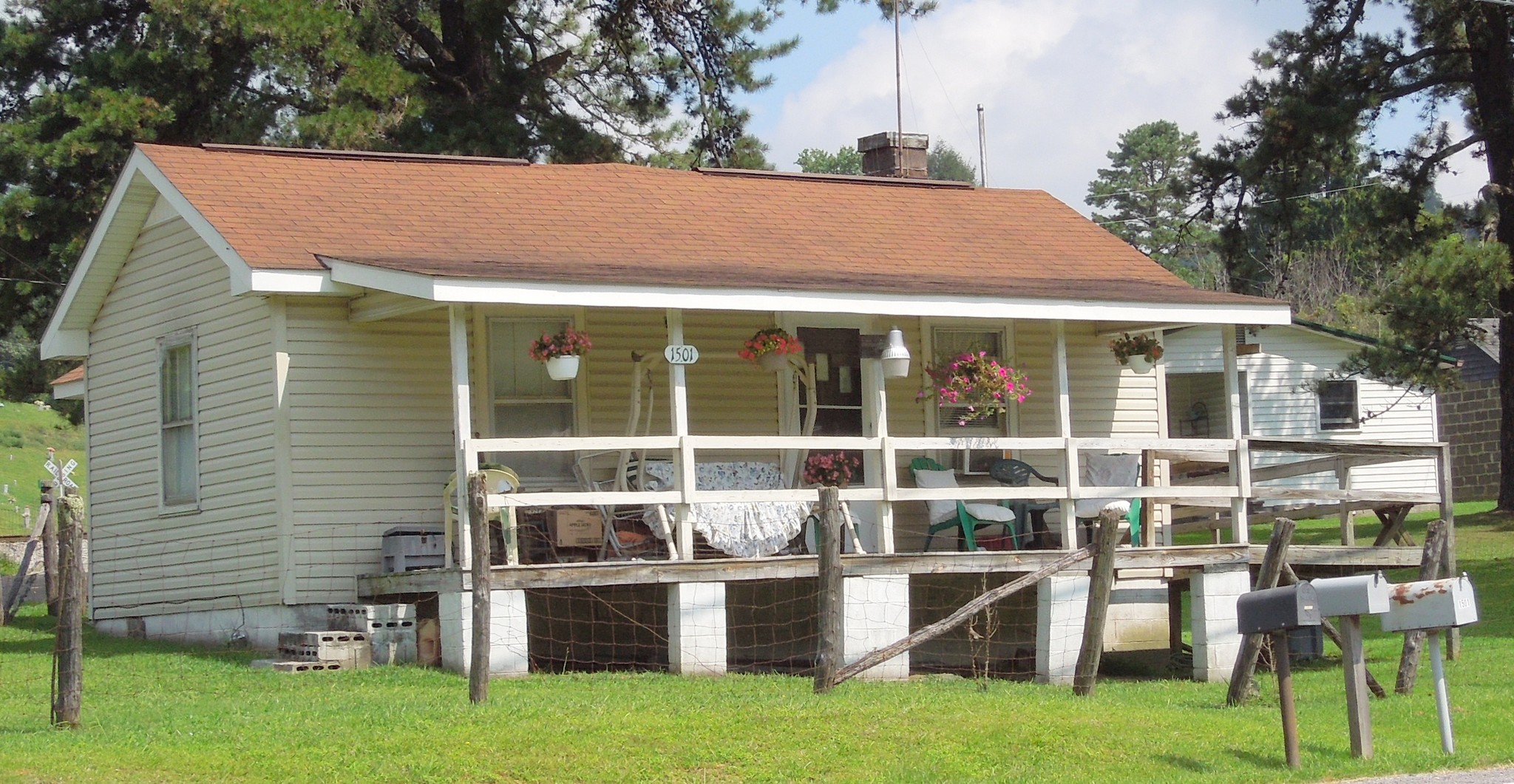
[990,459,1059,549]
[443,463,518,566]
[1076,452,1141,547]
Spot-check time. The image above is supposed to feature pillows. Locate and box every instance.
[1085,452,1139,486]
[913,469,965,520]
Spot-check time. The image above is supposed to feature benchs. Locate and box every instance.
[643,462,814,557]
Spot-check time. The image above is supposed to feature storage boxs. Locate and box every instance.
[546,509,604,547]
[380,526,445,573]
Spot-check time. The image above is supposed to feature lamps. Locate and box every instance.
[881,325,911,380]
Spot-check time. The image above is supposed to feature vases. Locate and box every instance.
[759,350,788,372]
[546,355,580,381]
[1129,354,1154,374]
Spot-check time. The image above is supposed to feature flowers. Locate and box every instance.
[737,328,803,358]
[1108,333,1164,364]
[802,450,859,486]
[527,325,592,363]
[914,348,1033,427]
